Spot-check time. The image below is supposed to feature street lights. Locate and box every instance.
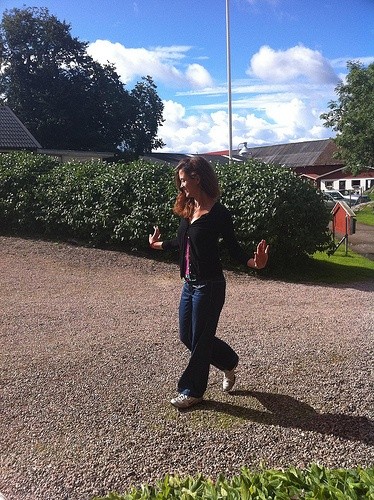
[235,140,250,163]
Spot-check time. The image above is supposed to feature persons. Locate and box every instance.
[149,159,270,409]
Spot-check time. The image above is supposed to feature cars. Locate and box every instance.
[317,191,355,213]
[326,189,369,204]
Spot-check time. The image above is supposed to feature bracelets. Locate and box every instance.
[151,242,157,249]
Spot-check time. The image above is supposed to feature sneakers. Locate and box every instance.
[170,393,203,409]
[222,356,239,391]
